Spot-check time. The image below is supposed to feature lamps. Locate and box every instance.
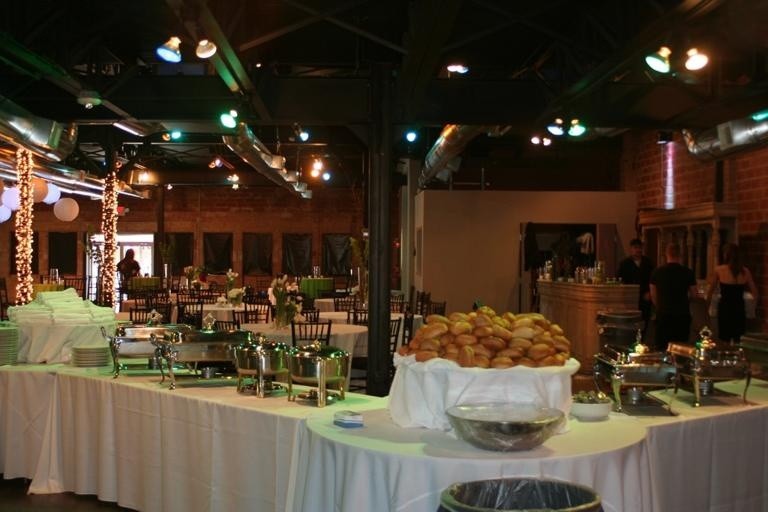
[644,19,710,77]
[155,5,217,66]
[547,111,587,138]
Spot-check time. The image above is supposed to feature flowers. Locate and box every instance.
[183,265,198,278]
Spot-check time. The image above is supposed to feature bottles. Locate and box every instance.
[573,258,606,284]
[539,260,551,279]
[50,268,58,280]
[313,266,321,277]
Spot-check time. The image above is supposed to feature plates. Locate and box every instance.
[72,345,110,367]
[0,327,18,365]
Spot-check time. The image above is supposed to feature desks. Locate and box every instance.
[2,355,768,512]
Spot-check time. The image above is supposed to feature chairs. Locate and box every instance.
[65,268,448,381]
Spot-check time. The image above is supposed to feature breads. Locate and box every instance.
[396,306,570,368]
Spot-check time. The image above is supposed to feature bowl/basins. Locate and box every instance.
[572,399,613,420]
[448,403,563,450]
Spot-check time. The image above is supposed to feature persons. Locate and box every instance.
[648,242,699,354]
[115,248,143,281]
[618,238,655,346]
[702,244,760,352]
